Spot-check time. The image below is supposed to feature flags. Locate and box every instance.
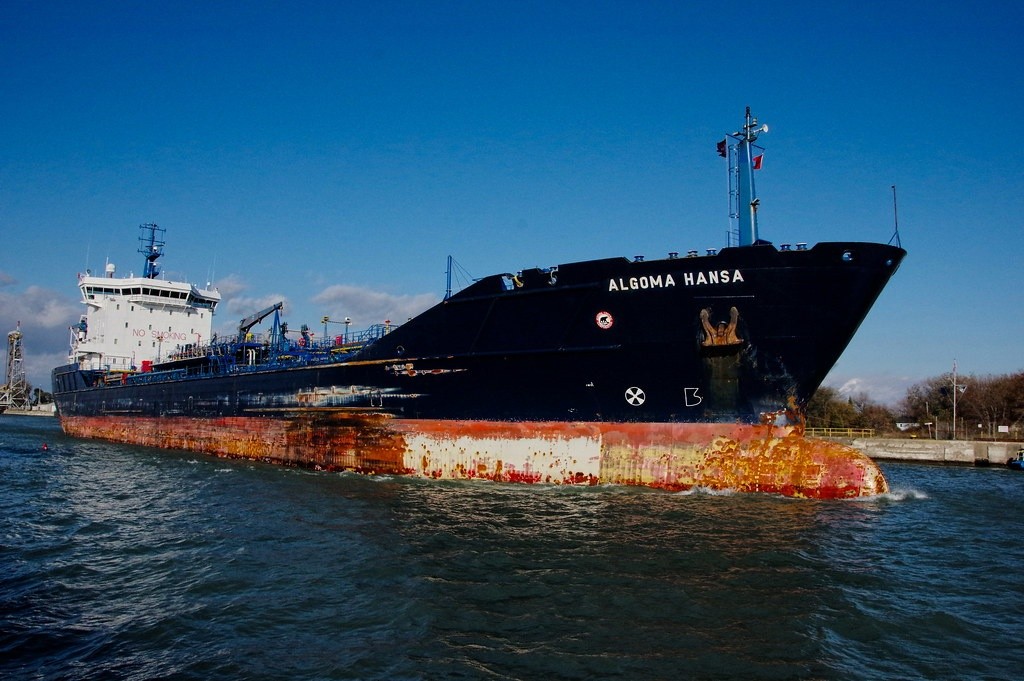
[753,155,763,169]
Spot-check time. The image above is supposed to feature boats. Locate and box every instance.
[1011,460,1024,470]
[51,106,908,501]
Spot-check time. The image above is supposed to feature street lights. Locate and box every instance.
[953,358,957,440]
[38,384,42,404]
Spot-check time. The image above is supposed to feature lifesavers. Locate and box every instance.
[298,338,306,346]
